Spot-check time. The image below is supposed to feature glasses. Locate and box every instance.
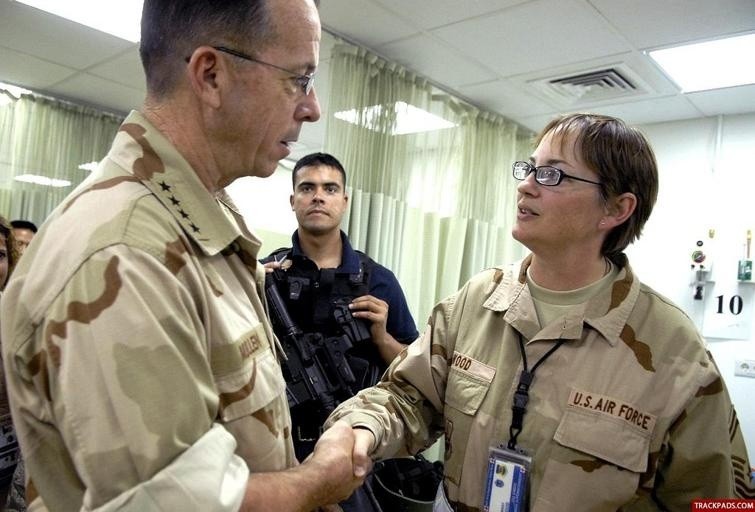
[184,45,315,95]
[513,160,605,187]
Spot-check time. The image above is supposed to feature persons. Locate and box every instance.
[1,0,376,510]
[248,150,422,508]
[0,218,37,510]
[320,111,755,512]
[7,219,37,255]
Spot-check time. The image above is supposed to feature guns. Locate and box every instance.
[263,272,384,511]
